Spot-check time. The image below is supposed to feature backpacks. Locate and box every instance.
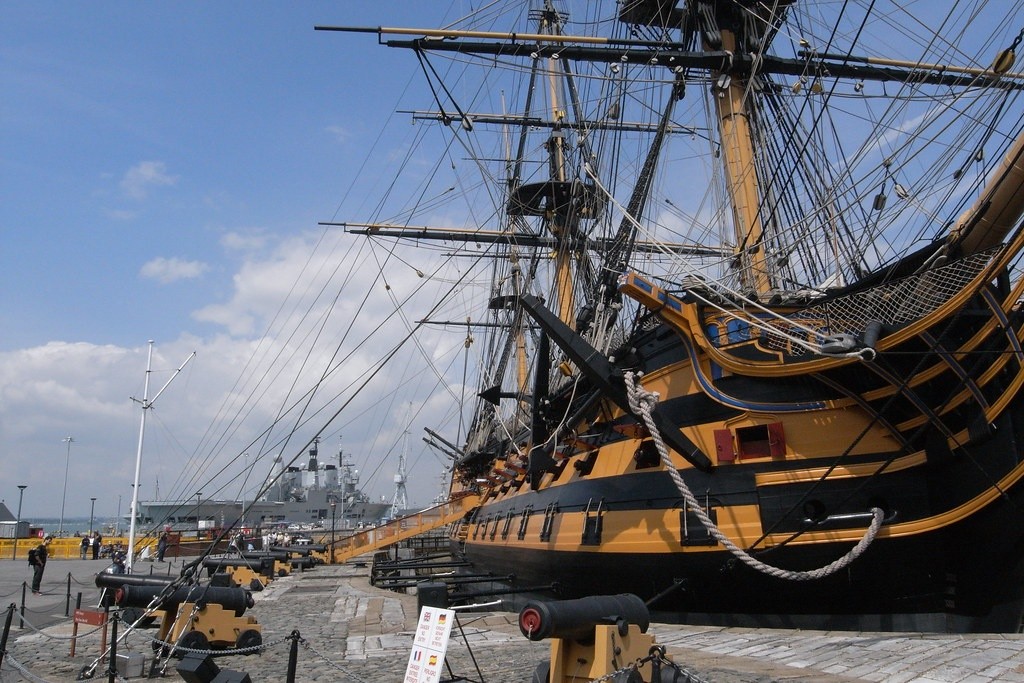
[28,549,37,566]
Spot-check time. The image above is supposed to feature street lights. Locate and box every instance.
[195,492,202,532]
[12,485,28,560]
[326,491,337,567]
[59,437,76,537]
[87,497,97,536]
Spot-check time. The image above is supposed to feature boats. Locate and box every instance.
[312,0,1024,620]
[124,429,394,532]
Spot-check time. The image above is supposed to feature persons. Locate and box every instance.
[101,541,124,573]
[31,536,52,596]
[92,531,102,560]
[158,531,168,562]
[81,535,90,561]
[267,531,291,546]
[229,532,245,553]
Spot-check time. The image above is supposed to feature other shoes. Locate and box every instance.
[34,592,42,595]
[158,560,165,562]
